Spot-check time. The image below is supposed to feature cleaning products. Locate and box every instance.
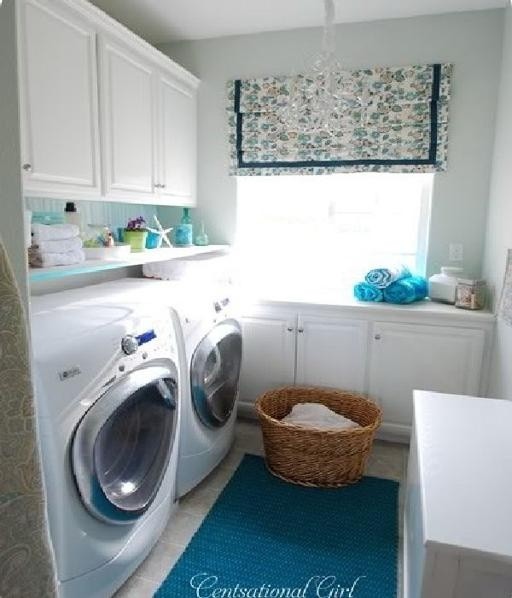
[176,207,194,245]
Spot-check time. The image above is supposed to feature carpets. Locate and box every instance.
[151,449,402,597]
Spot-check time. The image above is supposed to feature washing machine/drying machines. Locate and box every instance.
[151,251,243,498]
[28,277,182,597]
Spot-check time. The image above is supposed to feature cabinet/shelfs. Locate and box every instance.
[96,8,200,207]
[0,1,101,198]
[236,299,374,422]
[402,389,511,597]
[371,306,492,444]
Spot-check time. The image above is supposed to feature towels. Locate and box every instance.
[28,224,86,267]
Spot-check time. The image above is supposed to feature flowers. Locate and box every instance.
[125,214,147,231]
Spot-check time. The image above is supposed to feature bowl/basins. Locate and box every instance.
[117,227,164,249]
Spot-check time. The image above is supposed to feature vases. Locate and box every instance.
[124,231,147,252]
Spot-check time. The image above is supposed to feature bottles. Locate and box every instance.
[453,277,488,311]
[195,221,209,246]
[181,207,193,245]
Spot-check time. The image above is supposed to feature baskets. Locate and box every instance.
[257,386,383,488]
